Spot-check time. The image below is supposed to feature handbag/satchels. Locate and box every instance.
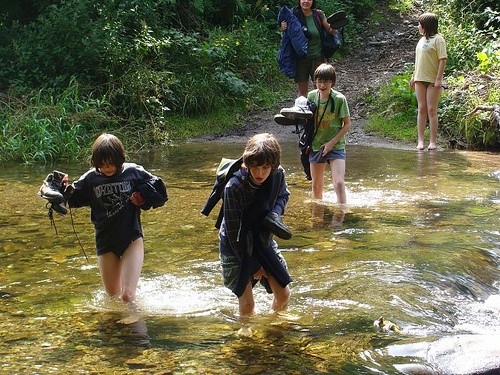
[313,9,342,58]
[298,131,313,156]
[201,156,246,229]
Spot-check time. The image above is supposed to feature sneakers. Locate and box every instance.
[51,184,75,217]
[36,171,64,204]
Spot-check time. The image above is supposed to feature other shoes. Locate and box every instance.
[258,219,275,249]
[331,18,349,28]
[274,114,307,126]
[327,11,346,24]
[263,212,292,240]
[280,97,313,119]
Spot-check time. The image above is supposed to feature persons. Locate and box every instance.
[307,63,351,204]
[218,133,294,314]
[62,133,169,303]
[281,0,337,99]
[410,13,447,150]
